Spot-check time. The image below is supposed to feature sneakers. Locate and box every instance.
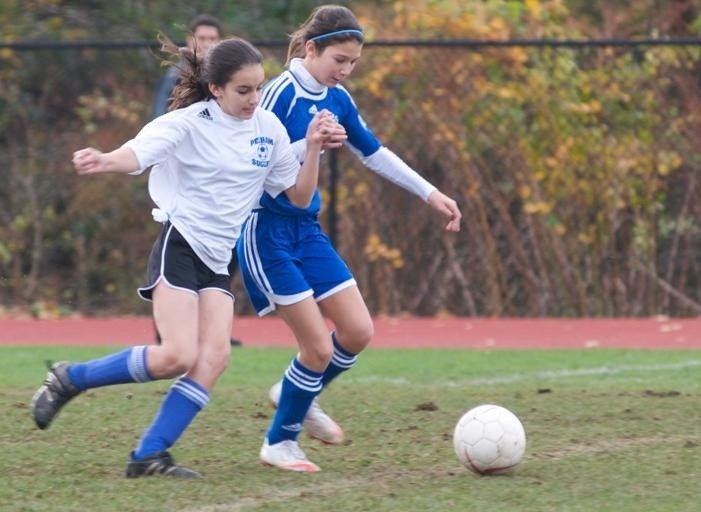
[31,360,80,430]
[260,437,323,473]
[268,379,344,444]
[125,449,202,480]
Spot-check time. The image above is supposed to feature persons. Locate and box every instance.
[237,6,463,472]
[30,32,338,482]
[153,13,240,346]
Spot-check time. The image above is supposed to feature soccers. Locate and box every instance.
[454,405,526,475]
